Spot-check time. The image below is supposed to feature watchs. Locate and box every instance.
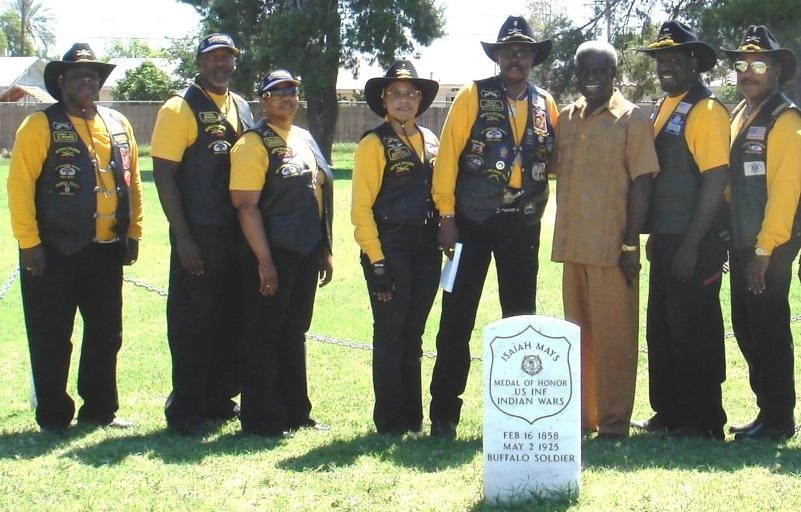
[753,248,771,258]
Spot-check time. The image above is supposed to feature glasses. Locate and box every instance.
[379,85,422,100]
[264,86,299,97]
[732,58,781,75]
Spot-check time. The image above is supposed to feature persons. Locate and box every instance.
[549,39,663,442]
[350,58,443,436]
[720,23,801,441]
[627,20,734,441]
[431,12,560,438]
[149,30,255,439]
[228,67,333,438]
[6,40,144,439]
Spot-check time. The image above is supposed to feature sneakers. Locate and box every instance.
[429,416,457,440]
[213,404,240,417]
[78,410,133,427]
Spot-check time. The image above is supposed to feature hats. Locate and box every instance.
[257,70,302,94]
[479,15,553,67]
[196,31,239,60]
[43,43,117,104]
[632,20,717,73]
[718,23,797,86]
[363,60,439,120]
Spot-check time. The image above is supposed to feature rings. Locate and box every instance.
[437,246,442,251]
[447,248,454,253]
[25,265,31,269]
[266,283,272,288]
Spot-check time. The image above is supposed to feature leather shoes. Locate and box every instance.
[729,420,787,444]
[629,416,725,443]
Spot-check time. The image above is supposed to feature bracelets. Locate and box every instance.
[619,241,638,253]
